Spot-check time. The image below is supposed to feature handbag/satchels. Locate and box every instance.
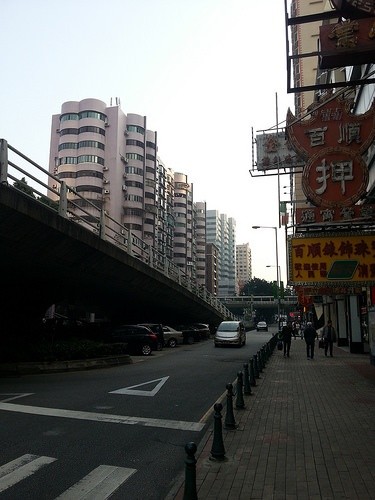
[277,342,283,350]
[318,340,324,349]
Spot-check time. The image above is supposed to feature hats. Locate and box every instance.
[327,320,332,323]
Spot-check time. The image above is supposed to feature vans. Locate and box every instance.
[213,320,246,348]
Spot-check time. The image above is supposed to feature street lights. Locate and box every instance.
[266,265,282,281]
[252,225,281,330]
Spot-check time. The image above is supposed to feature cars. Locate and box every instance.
[105,319,211,355]
[256,321,269,331]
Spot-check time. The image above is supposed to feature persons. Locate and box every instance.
[321,320,336,357]
[304,322,318,359]
[279,321,293,357]
